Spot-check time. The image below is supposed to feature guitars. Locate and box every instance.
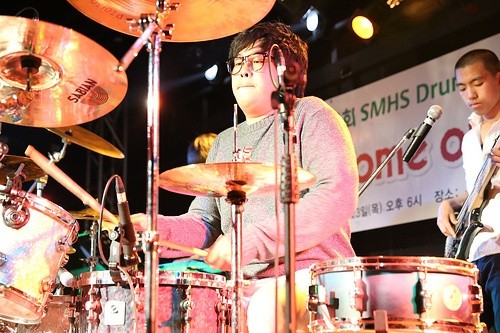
[444,131,500,260]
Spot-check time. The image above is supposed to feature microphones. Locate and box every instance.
[402,105,443,163]
[115,176,139,266]
[274,47,289,122]
[58,268,74,286]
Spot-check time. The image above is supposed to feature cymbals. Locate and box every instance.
[68,0,277,43]
[158,161,317,197]
[1,14,129,126]
[44,125,127,159]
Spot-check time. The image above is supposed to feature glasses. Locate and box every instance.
[226,52,273,74]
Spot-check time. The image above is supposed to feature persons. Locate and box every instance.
[118,22,359,333]
[436,49,500,333]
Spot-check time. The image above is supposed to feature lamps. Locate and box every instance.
[348,0,392,41]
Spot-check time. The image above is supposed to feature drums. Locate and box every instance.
[306,255,490,333]
[73,270,250,332]
[0,184,80,324]
